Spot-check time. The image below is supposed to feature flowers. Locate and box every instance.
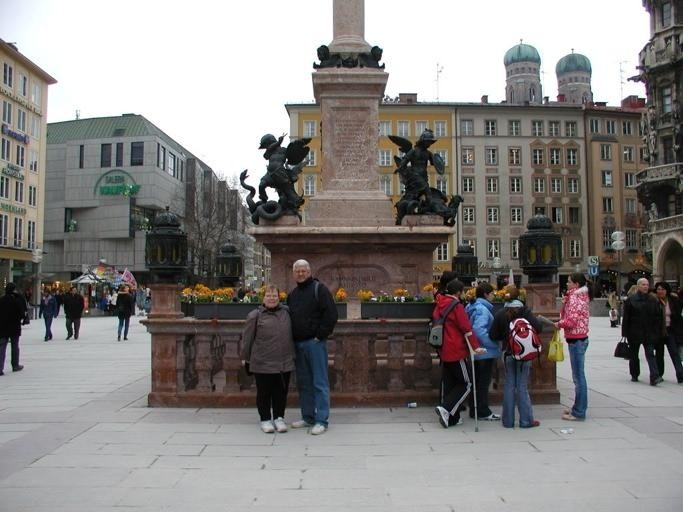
[179,281,347,303]
[358,282,527,303]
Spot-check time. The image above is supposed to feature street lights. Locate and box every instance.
[492,257,501,287]
[29,244,43,319]
[611,230,625,299]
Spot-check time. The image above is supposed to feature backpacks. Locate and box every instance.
[509,318,544,361]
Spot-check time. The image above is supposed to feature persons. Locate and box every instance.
[429,270,468,411]
[434,284,489,428]
[232,281,263,303]
[607,289,621,326]
[608,306,619,328]
[393,129,445,207]
[114,281,135,341]
[62,285,85,340]
[39,289,58,341]
[0,282,26,376]
[627,284,640,299]
[464,280,502,422]
[652,282,683,384]
[622,277,666,386]
[38,281,151,321]
[489,285,541,429]
[20,287,31,325]
[554,272,593,422]
[240,284,295,434]
[286,259,338,435]
[258,132,297,203]
[653,280,683,298]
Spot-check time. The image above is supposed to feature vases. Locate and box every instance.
[360,303,526,318]
[194,304,347,320]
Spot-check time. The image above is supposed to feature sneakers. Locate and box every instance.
[632,376,664,385]
[291,420,313,428]
[261,420,274,433]
[478,413,501,420]
[532,419,539,426]
[117,336,128,340]
[435,405,449,427]
[45,335,78,339]
[561,408,585,420]
[312,424,327,435]
[0,365,24,375]
[273,417,287,433]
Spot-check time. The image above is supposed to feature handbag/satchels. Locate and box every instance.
[425,319,444,347]
[547,340,564,362]
[615,342,630,358]
[246,362,251,376]
